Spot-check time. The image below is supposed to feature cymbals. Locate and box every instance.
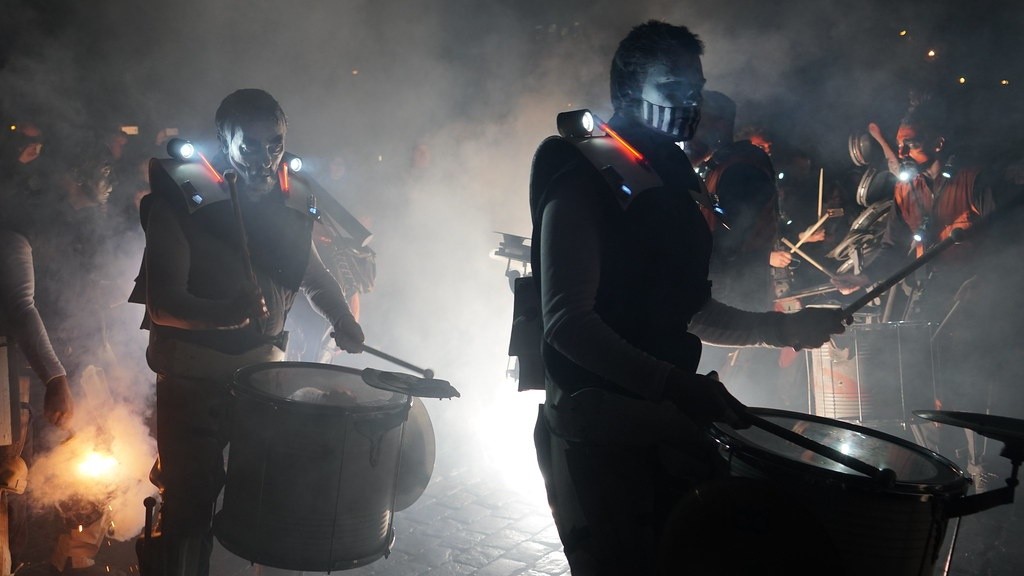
[912,409,1024,465]
[394,396,437,514]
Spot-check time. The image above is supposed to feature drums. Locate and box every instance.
[803,320,942,430]
[703,403,972,576]
[849,196,894,256]
[835,249,881,276]
[847,125,881,166]
[213,359,416,575]
[856,163,890,209]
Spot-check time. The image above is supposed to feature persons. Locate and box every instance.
[0,112,205,576]
[127,87,378,576]
[682,84,1007,494]
[382,140,434,193]
[529,20,853,576]
